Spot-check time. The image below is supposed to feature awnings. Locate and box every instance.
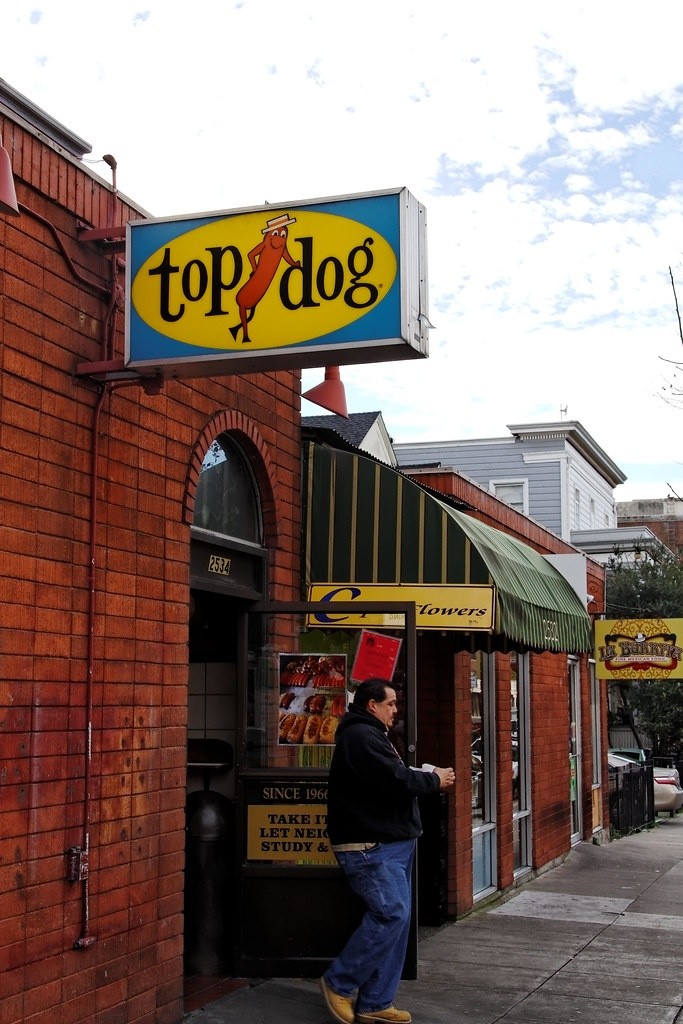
[301,442,590,653]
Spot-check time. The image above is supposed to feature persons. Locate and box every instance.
[320,678,455,1024]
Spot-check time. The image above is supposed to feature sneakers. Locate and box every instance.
[320,976,354,1024]
[357,1006,412,1023]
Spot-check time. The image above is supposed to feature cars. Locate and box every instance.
[608,752,683,825]
